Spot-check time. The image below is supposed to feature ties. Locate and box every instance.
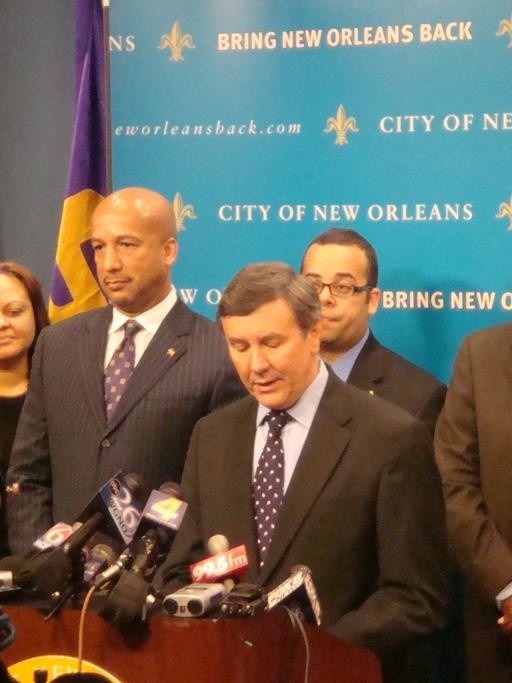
[103,319,144,426]
[251,411,292,571]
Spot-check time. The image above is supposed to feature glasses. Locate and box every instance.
[311,279,372,298]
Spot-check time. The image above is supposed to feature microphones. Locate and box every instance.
[43,544,117,623]
[0,520,83,592]
[39,468,149,602]
[208,533,236,599]
[89,545,131,591]
[108,481,189,624]
[288,564,313,587]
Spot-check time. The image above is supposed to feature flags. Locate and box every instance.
[45,1,110,329]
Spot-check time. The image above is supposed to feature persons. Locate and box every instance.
[299,224,450,437]
[6,185,253,565]
[430,319,511,681]
[152,259,455,682]
[0,259,54,560]
